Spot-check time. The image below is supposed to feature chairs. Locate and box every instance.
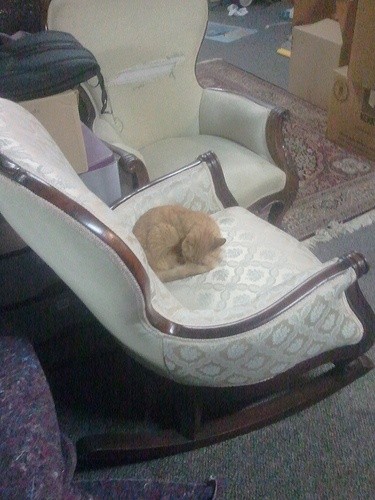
[0,98,375,458]
[40,1,300,231]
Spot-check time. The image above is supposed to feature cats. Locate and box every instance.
[132,205,226,283]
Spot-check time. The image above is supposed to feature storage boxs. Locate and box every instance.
[287,17,344,109]
[76,120,121,207]
[326,65,374,160]
[346,0,375,90]
[13,90,89,176]
